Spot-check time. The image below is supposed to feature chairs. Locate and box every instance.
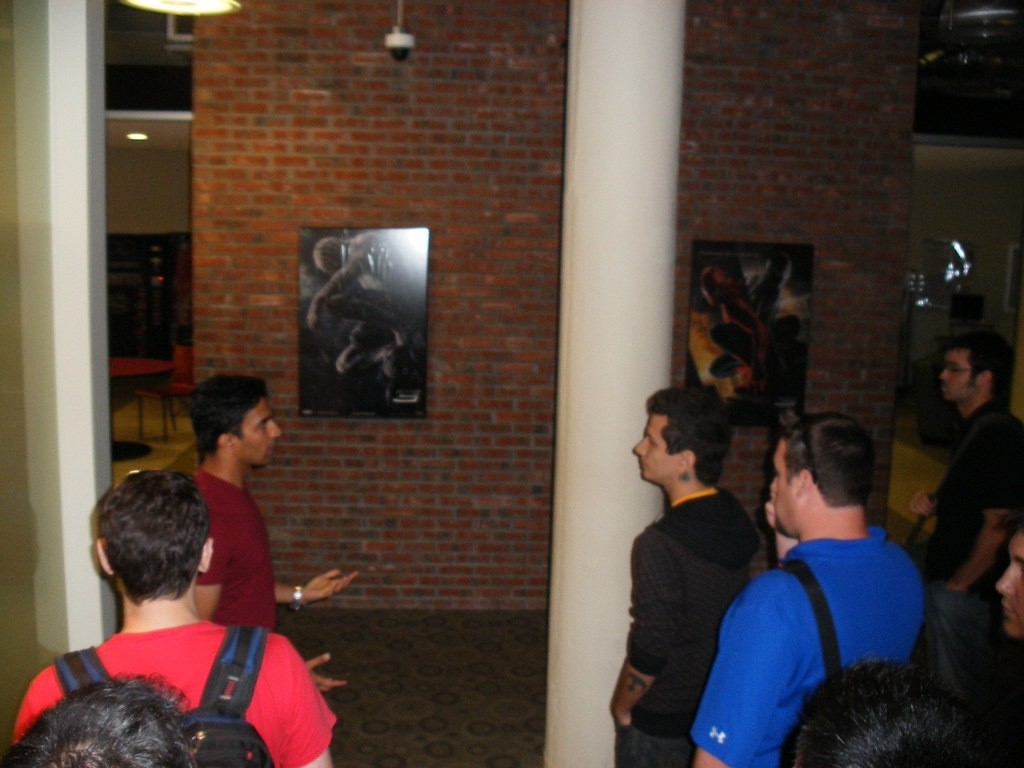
[133,345,195,442]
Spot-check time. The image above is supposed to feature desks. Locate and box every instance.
[109,355,176,462]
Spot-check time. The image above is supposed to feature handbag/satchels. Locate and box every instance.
[901,541,928,580]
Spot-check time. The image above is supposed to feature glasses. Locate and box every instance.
[944,363,978,374]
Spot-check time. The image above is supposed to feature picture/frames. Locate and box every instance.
[1003,243,1024,313]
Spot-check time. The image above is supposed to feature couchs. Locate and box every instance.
[909,346,1016,447]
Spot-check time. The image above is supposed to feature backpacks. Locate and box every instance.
[53,624,275,768]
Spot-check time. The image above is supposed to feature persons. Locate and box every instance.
[701,254,798,402]
[0,374,357,768]
[307,234,426,417]
[609,327,1024,768]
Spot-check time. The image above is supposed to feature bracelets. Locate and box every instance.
[289,583,305,610]
